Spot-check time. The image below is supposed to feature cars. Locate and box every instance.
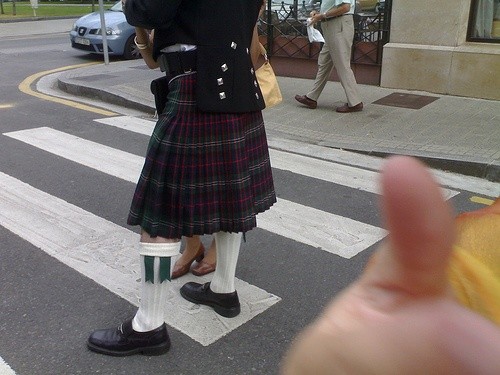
[375,1,386,15]
[266,0,322,34]
[69,0,145,59]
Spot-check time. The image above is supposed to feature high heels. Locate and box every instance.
[171,242,204,278]
[192,258,218,275]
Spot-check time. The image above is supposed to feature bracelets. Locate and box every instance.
[323,13,327,18]
[137,38,151,49]
[134,34,150,47]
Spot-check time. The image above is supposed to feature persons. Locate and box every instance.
[86,0,276,358]
[132,10,267,276]
[281,153,500,375]
[294,0,363,112]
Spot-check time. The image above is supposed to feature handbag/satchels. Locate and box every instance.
[253,42,283,107]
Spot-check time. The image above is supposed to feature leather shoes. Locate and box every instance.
[87,319,171,356]
[180,281,240,319]
[295,94,317,108]
[335,101,363,113]
